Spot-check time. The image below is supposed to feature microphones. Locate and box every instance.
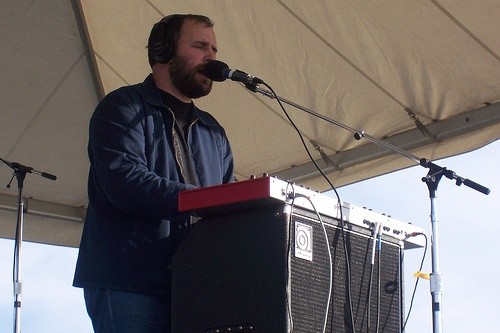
[205,60,263,84]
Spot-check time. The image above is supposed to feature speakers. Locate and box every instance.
[169,205,406,333]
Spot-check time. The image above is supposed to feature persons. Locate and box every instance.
[72,13,234,333]
